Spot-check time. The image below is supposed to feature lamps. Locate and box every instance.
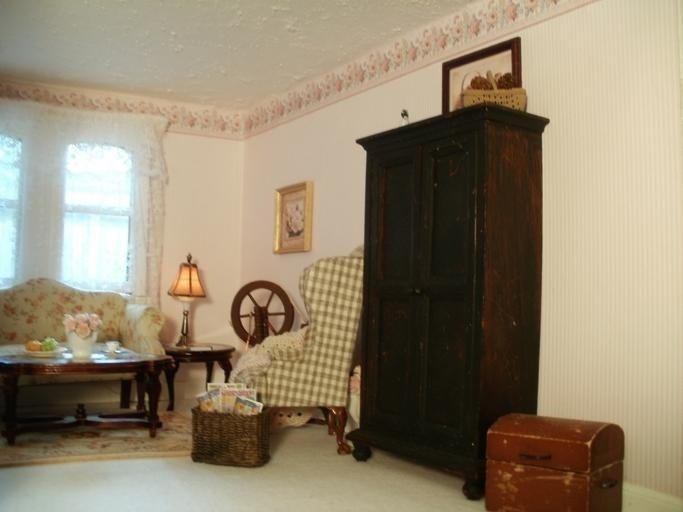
[167,253,208,346]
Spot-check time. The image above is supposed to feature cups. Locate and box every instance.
[105,339,121,355]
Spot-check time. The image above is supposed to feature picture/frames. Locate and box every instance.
[435,36,522,115]
[274,180,312,257]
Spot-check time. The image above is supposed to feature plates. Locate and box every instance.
[23,346,69,358]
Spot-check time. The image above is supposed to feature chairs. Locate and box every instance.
[227,252,364,458]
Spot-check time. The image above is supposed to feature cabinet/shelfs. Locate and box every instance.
[353,101,549,500]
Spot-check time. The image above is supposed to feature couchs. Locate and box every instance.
[1,275,169,438]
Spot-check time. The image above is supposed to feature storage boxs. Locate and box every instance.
[485,413,625,512]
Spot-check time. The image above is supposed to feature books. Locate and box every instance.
[195,383,263,415]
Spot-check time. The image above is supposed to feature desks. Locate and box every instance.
[0,340,170,447]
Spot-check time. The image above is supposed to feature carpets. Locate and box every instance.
[0,407,193,467]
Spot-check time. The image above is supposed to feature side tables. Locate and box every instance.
[164,343,235,411]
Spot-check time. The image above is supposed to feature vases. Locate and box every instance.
[68,332,97,363]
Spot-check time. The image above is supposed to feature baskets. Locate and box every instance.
[460,87,529,113]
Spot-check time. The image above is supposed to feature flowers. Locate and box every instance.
[60,310,108,338]
